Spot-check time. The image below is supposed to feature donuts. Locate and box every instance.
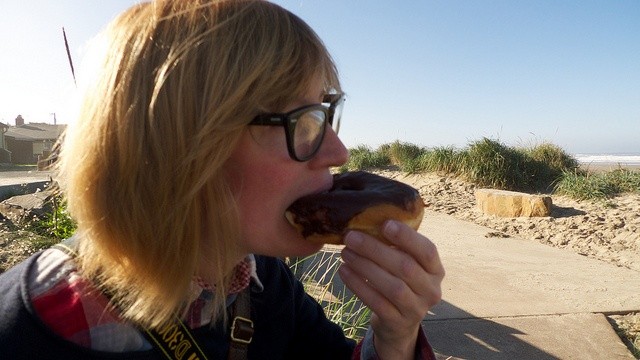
[283,171,424,245]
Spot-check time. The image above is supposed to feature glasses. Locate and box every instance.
[251,91,345,162]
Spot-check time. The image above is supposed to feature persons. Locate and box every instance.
[1,1,445,359]
